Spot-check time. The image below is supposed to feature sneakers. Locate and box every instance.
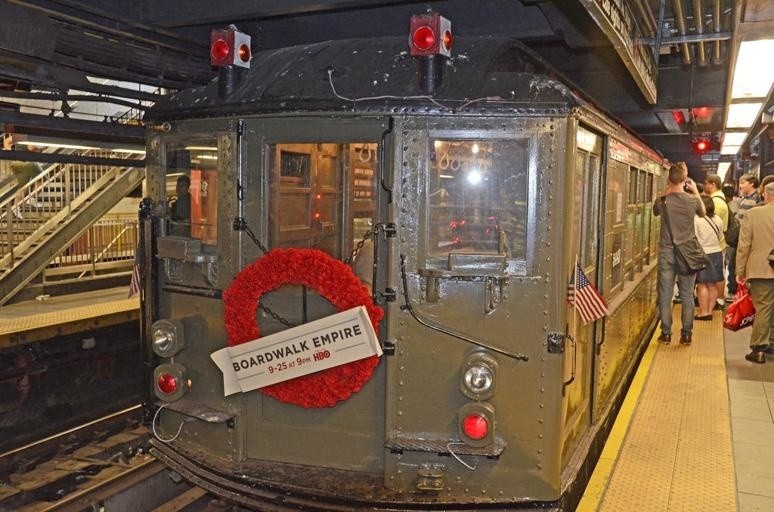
[680,335,692,345]
[673,293,735,320]
[658,331,671,345]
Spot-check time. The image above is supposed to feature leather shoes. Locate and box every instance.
[745,351,766,364]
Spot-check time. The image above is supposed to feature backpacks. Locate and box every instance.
[711,195,741,248]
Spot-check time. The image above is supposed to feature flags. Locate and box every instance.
[567,262,610,328]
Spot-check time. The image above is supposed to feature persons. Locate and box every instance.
[650,161,707,347]
[168,174,195,225]
[686,171,774,364]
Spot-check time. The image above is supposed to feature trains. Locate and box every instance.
[132,14,690,512]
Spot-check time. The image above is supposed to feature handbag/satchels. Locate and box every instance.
[673,235,710,275]
[723,282,756,331]
